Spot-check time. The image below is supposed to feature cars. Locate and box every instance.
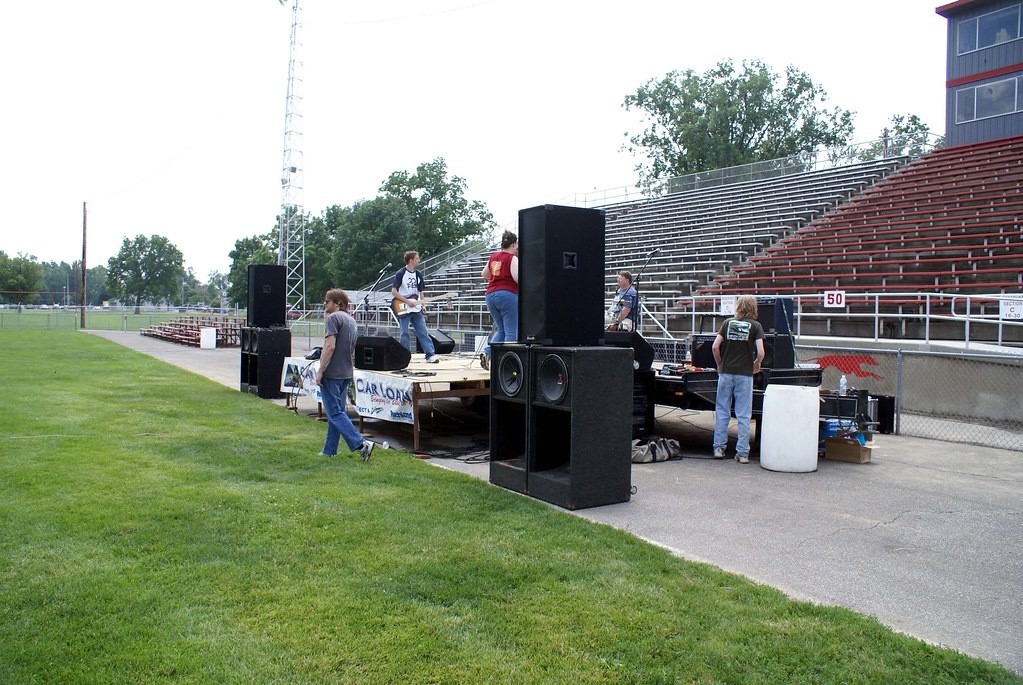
[176,305,229,313]
[0,304,109,310]
[286,311,301,320]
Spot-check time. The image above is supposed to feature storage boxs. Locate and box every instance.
[825,430,872,464]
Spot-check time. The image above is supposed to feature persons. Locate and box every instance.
[712,296,765,464]
[479,230,518,371]
[316,289,376,463]
[607,271,638,332]
[391,250,440,363]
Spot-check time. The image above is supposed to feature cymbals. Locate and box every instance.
[604,298,631,303]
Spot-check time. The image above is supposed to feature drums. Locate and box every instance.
[604,309,618,324]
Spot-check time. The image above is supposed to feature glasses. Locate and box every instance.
[325,298,333,303]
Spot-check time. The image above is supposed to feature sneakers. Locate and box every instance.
[735,453,750,463]
[426,356,439,363]
[318,452,336,458]
[357,441,375,462]
[480,353,490,371]
[713,448,726,458]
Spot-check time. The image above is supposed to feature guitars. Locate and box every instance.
[392,292,458,319]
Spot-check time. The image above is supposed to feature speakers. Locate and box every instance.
[416,329,455,353]
[247,265,287,327]
[602,330,655,372]
[692,297,796,370]
[490,343,634,510]
[240,327,291,399]
[355,336,411,370]
[816,394,868,420]
[518,204,605,346]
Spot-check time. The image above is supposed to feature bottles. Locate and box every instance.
[383,441,389,449]
[839,375,848,396]
[685,351,692,370]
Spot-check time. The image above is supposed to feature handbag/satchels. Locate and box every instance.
[631,438,683,463]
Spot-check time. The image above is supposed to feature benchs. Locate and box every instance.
[361,133,1023,338]
[140,316,246,348]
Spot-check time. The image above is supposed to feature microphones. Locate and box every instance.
[647,248,661,256]
[380,263,392,274]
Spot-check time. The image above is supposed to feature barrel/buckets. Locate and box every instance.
[200,326,216,349]
[760,384,821,473]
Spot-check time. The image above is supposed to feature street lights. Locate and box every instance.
[75,197,100,326]
